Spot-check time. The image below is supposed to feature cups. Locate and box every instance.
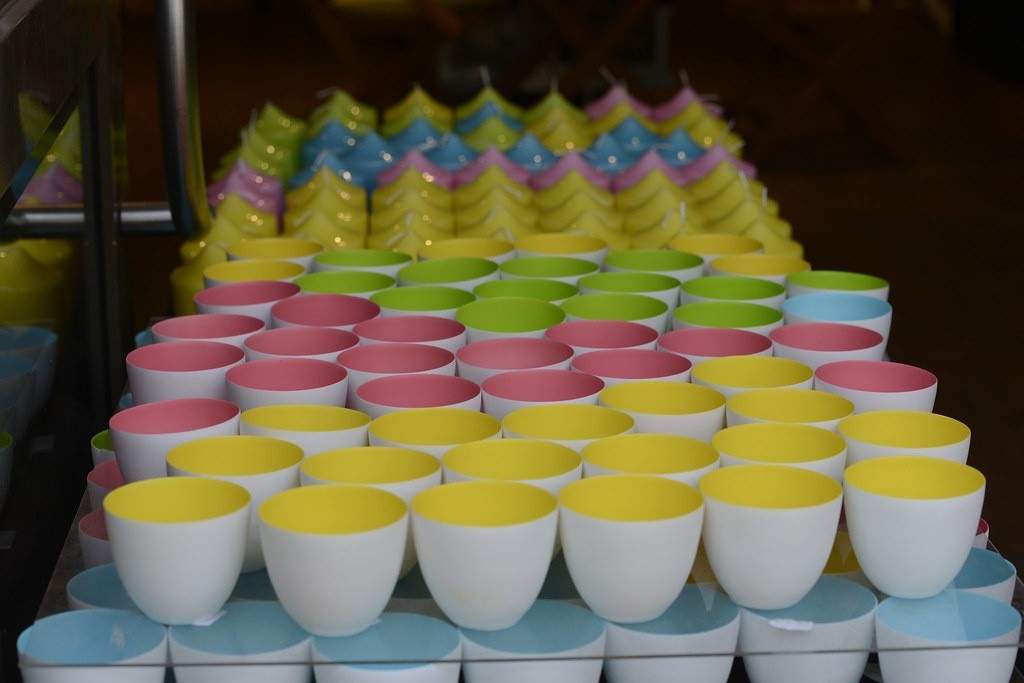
[0,322,61,509]
[14,237,1024,683]
